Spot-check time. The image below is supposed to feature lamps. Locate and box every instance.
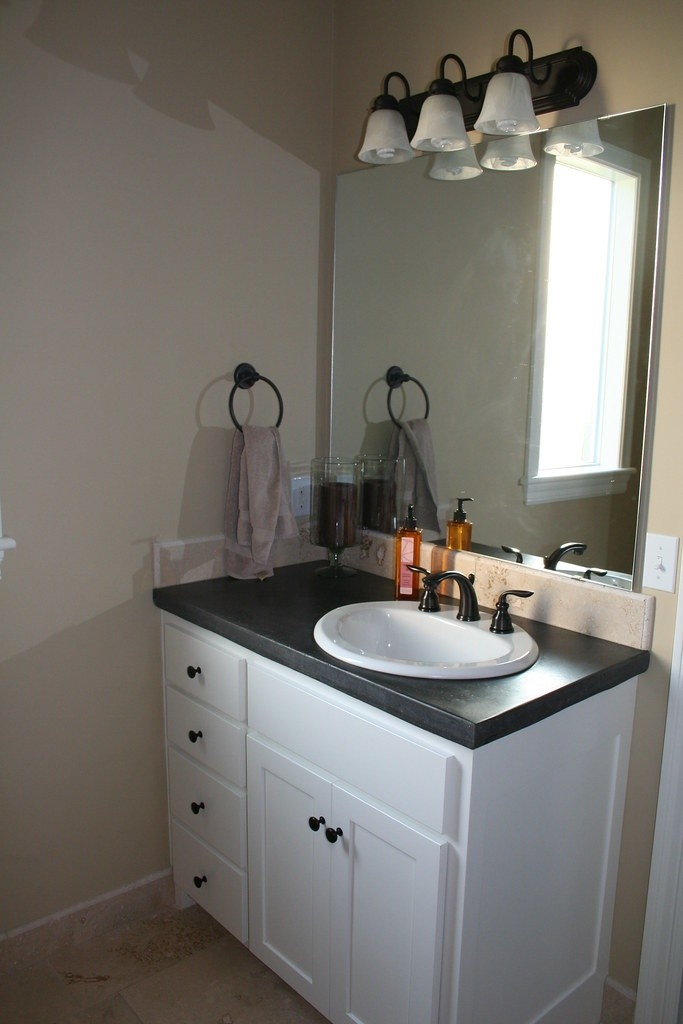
[357,72,419,165]
[543,119,604,157]
[428,146,483,180]
[478,135,538,171]
[410,54,482,152]
[474,30,551,136]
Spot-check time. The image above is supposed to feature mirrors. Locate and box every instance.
[328,102,666,591]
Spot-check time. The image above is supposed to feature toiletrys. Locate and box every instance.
[445,497,474,551]
[393,504,423,601]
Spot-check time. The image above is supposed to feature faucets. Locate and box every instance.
[543,542,587,570]
[422,570,480,622]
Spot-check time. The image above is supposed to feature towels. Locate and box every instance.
[387,418,442,534]
[222,424,299,580]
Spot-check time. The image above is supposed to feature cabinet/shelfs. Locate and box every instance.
[160,609,250,955]
[248,646,638,1024]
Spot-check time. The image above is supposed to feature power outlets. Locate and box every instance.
[643,533,680,594]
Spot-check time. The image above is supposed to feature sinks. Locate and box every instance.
[313,603,540,680]
[559,570,632,590]
